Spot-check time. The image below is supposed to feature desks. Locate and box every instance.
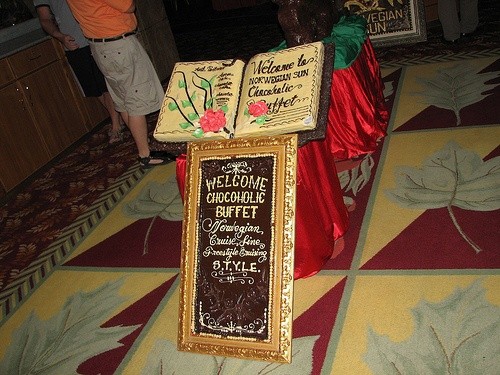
[175,14,390,283]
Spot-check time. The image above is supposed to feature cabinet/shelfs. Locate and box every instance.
[0,0,181,194]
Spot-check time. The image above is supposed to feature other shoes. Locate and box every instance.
[441,36,457,45]
[461,33,471,37]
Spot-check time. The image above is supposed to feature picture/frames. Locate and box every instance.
[177,134,299,363]
[331,0,427,48]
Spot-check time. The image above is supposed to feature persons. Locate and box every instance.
[66,0,165,165]
[33,0,124,145]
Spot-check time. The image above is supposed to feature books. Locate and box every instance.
[153,40,336,144]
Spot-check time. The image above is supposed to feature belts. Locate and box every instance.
[84,31,136,42]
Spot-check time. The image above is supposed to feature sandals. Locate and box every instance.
[136,151,171,169]
[120,124,126,130]
[109,129,123,146]
